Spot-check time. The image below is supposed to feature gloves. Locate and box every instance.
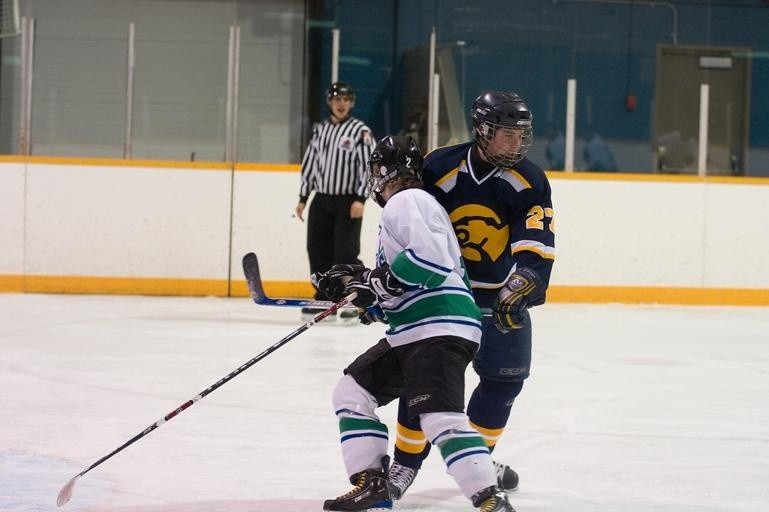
[491,266,546,335]
[358,310,379,326]
[310,262,419,309]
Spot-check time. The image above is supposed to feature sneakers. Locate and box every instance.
[472,485,517,512]
[490,457,518,490]
[301,306,359,318]
[322,468,393,511]
[385,460,432,501]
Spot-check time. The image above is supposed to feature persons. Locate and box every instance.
[311,134,515,512]
[544,122,623,173]
[378,89,554,500]
[296,81,377,318]
[651,123,699,174]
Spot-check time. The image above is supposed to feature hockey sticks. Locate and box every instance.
[243,253,492,317]
[56,291,358,506]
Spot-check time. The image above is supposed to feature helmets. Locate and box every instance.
[325,80,355,108]
[470,89,534,172]
[366,135,426,208]
